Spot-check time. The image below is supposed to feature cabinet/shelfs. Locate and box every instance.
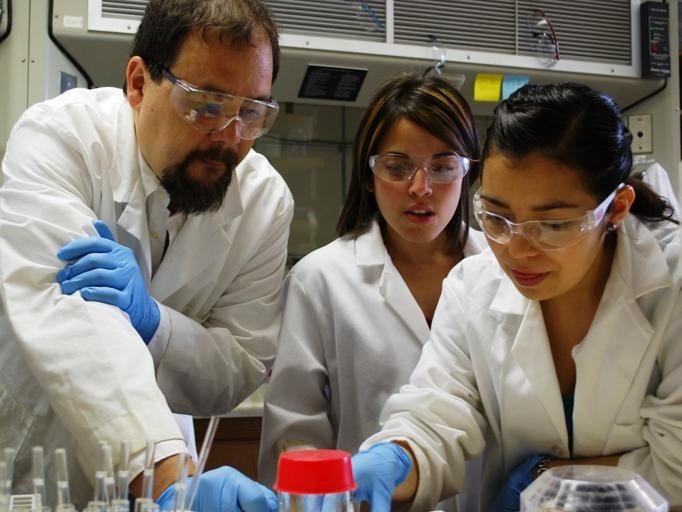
[194,412,263,482]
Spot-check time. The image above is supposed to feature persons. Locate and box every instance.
[257,74,491,512]
[300,79,682,512]
[0,0,295,511]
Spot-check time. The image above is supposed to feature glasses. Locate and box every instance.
[368,155,472,185]
[144,58,280,140]
[471,183,625,250]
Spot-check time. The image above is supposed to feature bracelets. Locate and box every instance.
[536,454,553,476]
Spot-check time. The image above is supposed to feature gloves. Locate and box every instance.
[302,443,411,512]
[156,464,279,512]
[55,220,160,346]
[492,453,545,511]
